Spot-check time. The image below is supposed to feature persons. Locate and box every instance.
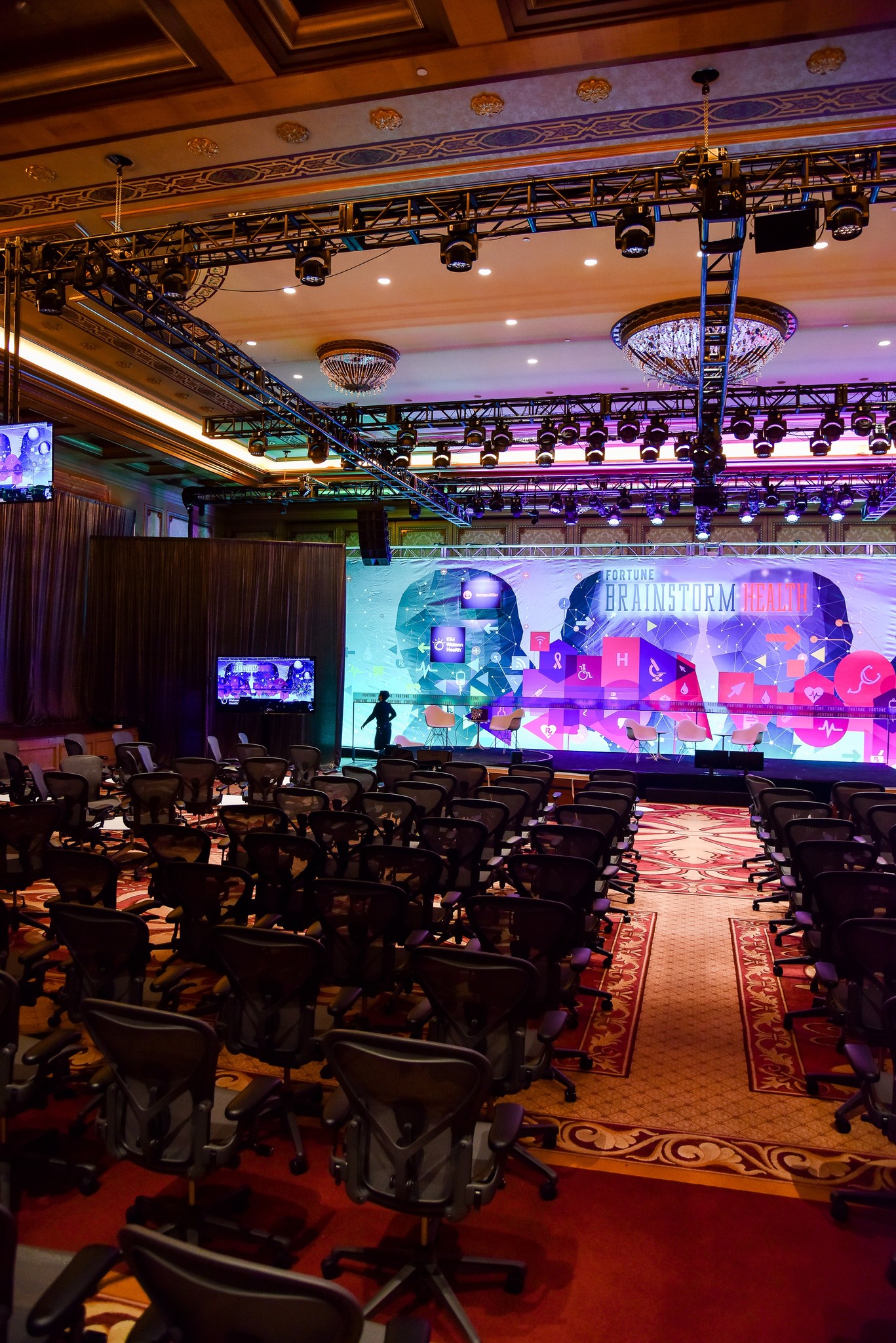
[361,690,396,769]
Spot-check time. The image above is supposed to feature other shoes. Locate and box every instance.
[373,765,377,769]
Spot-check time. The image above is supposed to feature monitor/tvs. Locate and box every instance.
[694,750,729,774]
[218,656,315,714]
[0,422,54,503]
[729,751,764,776]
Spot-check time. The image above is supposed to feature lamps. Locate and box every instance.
[36,67,896,541]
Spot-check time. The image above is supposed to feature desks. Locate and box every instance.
[465,720,489,750]
[713,733,733,750]
[646,732,671,760]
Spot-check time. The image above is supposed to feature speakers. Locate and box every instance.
[391,746,415,762]
[417,750,449,764]
[357,510,392,565]
[386,745,398,754]
[511,751,522,762]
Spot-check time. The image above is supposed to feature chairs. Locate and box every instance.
[741,773,896,1222]
[731,722,769,751]
[673,719,706,763]
[0,704,657,1343]
